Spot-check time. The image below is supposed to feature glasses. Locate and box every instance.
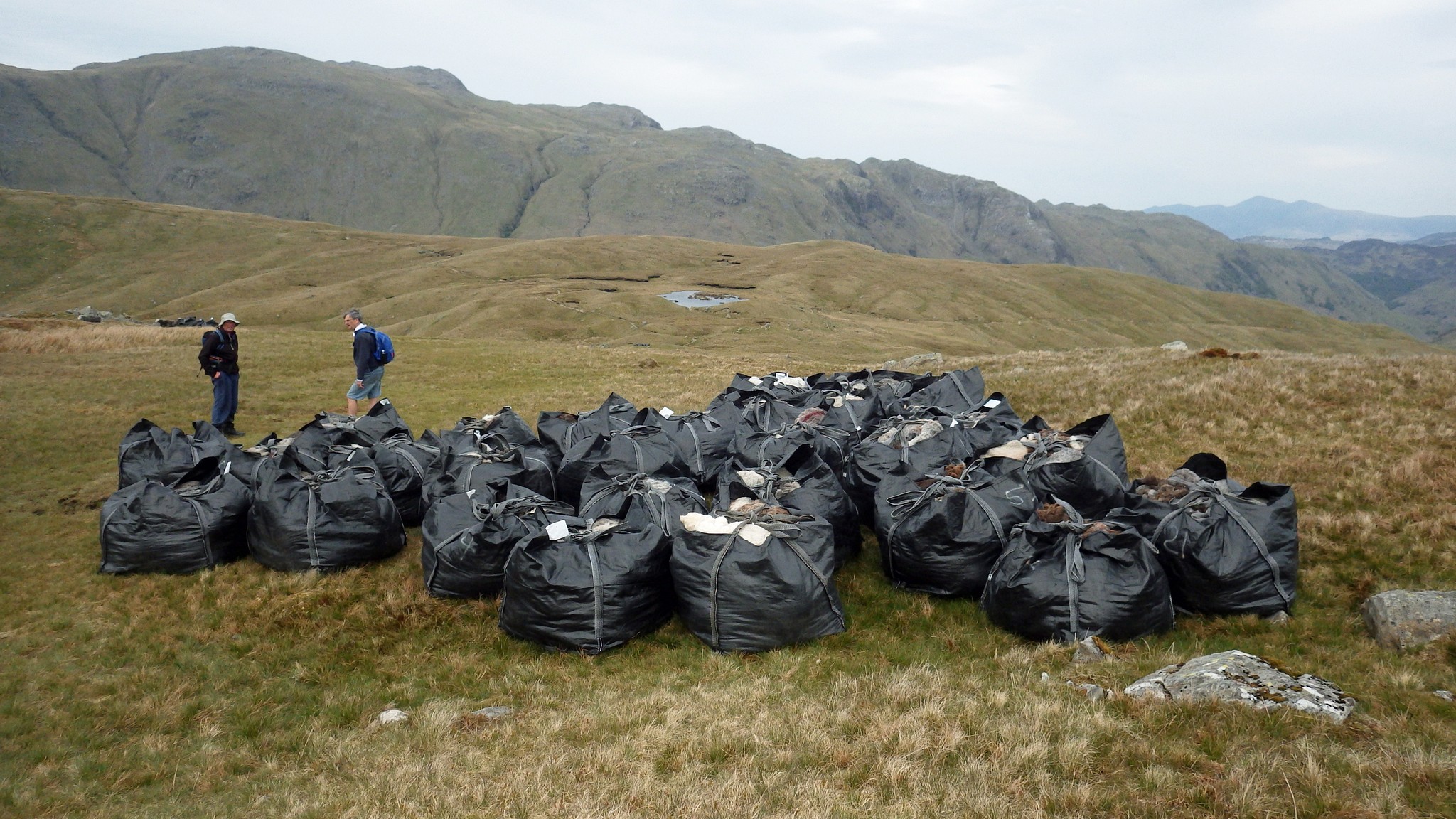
[345,319,355,322]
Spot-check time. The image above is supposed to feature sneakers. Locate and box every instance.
[226,429,245,437]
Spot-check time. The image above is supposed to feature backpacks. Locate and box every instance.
[359,325,394,363]
[199,330,224,371]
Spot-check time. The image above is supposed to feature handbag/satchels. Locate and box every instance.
[96,471,253,576]
[115,419,231,489]
[218,435,284,496]
[253,363,1303,662]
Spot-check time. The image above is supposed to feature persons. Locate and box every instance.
[198,313,245,442]
[343,309,384,420]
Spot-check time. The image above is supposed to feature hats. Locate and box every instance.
[218,313,240,327]
